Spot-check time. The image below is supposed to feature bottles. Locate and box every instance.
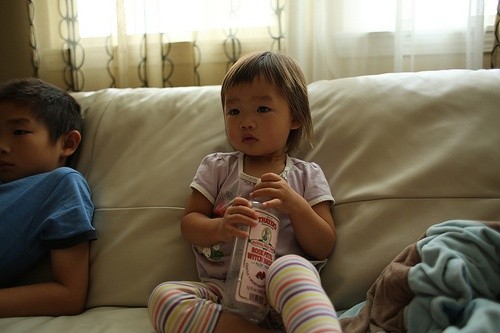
[223,178,279,322]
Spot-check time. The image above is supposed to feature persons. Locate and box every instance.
[147,50,341,333]
[0,78,97,319]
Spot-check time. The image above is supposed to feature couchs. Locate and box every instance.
[0,68,500,333]
